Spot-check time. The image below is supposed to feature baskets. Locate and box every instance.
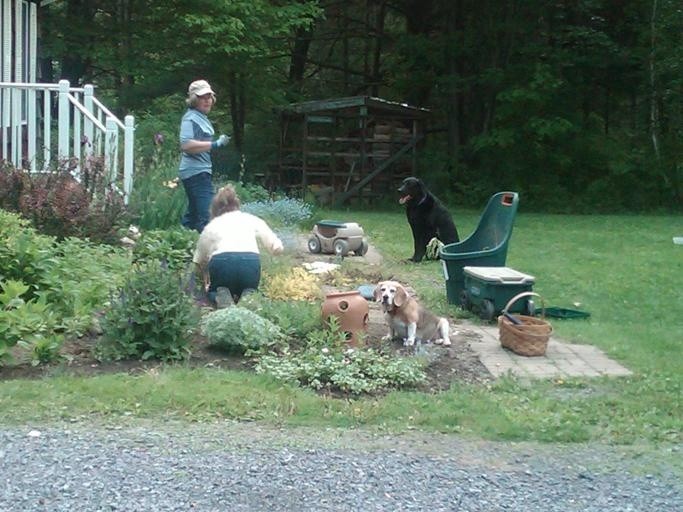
[498,290,553,356]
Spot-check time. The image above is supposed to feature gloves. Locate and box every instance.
[213,134,232,150]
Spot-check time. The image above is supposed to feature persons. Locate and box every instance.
[175,78,231,232]
[191,184,284,312]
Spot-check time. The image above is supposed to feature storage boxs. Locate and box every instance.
[460,265,536,320]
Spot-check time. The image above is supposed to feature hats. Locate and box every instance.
[189,80,216,97]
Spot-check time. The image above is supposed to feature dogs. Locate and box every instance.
[373,280,452,347]
[397,176,460,263]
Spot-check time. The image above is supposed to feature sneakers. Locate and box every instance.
[215,286,234,308]
[238,288,256,307]
